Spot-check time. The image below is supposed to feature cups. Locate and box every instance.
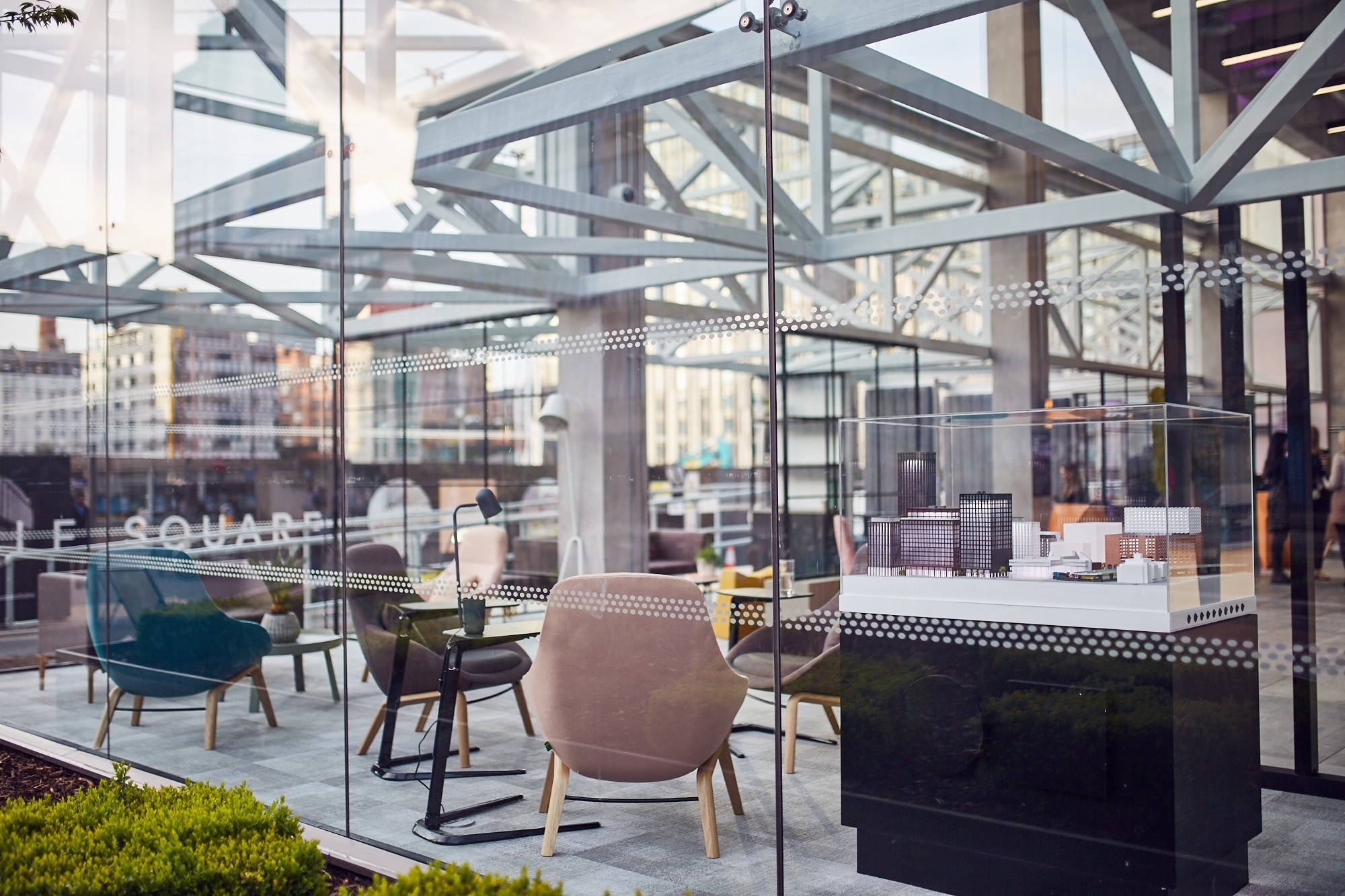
[462,597,485,635]
[779,559,795,596]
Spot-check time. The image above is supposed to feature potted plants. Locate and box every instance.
[246,548,308,643]
[694,547,720,575]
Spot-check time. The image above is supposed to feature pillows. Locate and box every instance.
[383,594,462,656]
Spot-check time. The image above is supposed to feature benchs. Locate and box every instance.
[513,529,715,602]
[37,557,273,704]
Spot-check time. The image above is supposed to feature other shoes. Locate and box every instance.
[1270,571,1291,584]
[1313,571,1331,582]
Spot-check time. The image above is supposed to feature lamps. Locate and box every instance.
[451,488,503,634]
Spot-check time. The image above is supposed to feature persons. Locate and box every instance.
[72,488,88,530]
[1053,461,1090,504]
[1262,424,1345,584]
[1125,454,1186,508]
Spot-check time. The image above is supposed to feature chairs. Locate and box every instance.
[726,543,870,775]
[85,548,278,752]
[521,572,749,858]
[361,524,508,684]
[340,542,534,769]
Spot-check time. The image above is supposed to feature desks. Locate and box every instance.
[216,630,343,712]
[412,618,599,846]
[712,586,837,759]
